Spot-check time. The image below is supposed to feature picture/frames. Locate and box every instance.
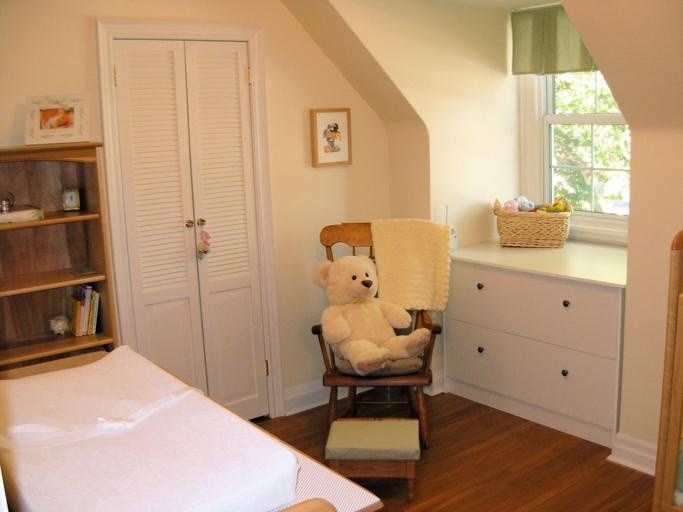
[25,94,90,146]
[309,107,352,169]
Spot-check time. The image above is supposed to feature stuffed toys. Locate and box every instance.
[312,254,433,378]
[503,196,571,212]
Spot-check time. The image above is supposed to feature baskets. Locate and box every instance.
[493,198,573,248]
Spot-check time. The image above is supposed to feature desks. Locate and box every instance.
[0,342,384,512]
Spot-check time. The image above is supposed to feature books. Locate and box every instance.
[0,204,45,224]
[66,284,100,337]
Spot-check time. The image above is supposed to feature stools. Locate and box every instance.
[324,416,421,502]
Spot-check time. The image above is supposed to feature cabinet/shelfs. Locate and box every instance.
[1,142,119,381]
[441,239,625,450]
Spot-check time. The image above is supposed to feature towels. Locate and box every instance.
[371,218,452,314]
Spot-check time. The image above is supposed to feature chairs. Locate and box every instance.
[312,222,443,449]
